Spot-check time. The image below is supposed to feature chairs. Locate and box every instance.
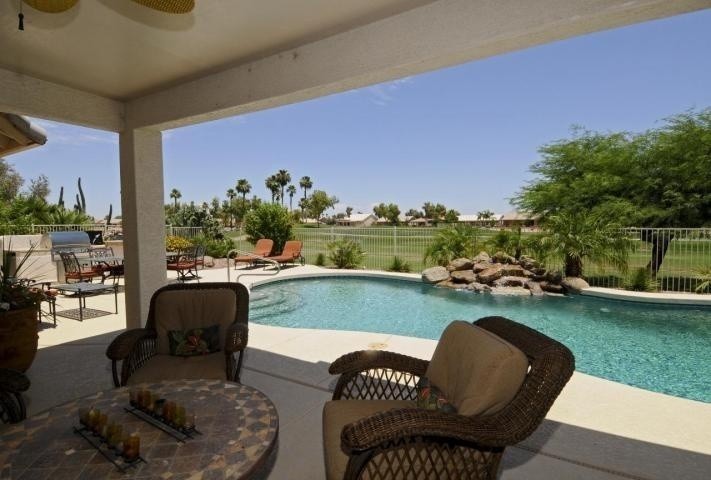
[105,283,249,388]
[323,315,575,479]
[16,239,305,327]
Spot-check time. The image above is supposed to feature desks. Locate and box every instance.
[0,377,281,480]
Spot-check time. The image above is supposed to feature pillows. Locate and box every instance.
[152,287,237,357]
[415,320,530,417]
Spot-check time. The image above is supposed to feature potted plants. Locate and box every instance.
[0,231,56,371]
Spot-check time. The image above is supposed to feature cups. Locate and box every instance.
[78,404,140,463]
[128,388,196,431]
